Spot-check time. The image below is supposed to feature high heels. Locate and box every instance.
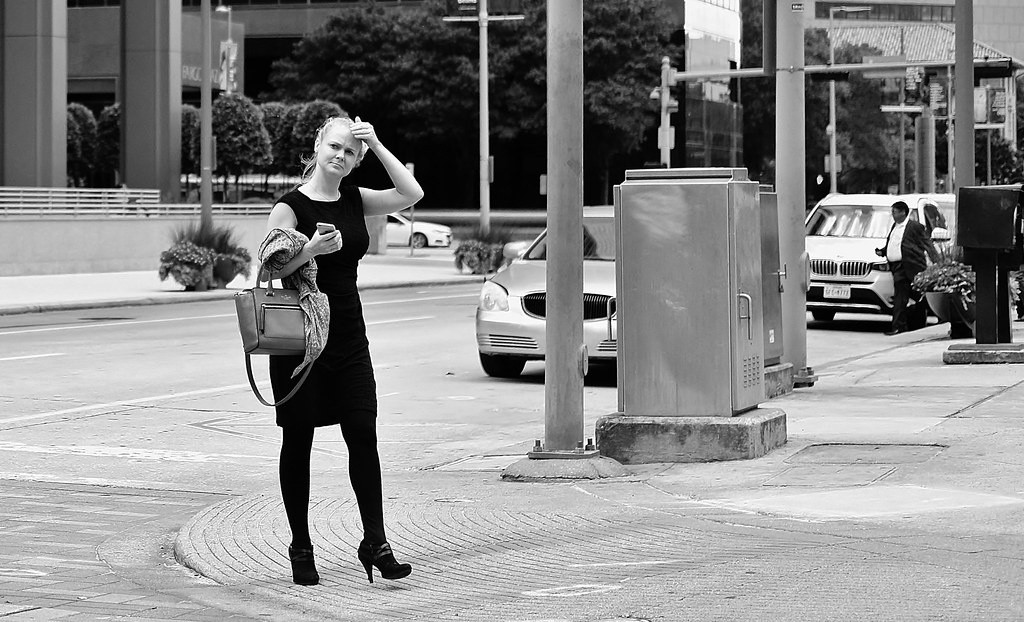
[288,542,320,586]
[357,539,412,583]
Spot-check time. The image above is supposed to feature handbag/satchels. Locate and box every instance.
[233,267,307,354]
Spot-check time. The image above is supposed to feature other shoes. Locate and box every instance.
[883,328,907,335]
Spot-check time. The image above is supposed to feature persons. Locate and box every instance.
[875,201,940,336]
[256,115,425,584]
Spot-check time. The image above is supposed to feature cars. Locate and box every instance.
[474,206,617,378]
[386,213,453,248]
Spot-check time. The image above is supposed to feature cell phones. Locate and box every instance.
[316,222,337,235]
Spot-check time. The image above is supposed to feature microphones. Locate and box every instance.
[649,92,659,101]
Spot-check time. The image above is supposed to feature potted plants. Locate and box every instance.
[158,216,251,292]
[910,258,976,341]
[450,223,517,274]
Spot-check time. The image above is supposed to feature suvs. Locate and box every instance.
[802,192,953,325]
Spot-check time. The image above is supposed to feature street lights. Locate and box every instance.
[215,3,234,93]
[826,5,872,190]
